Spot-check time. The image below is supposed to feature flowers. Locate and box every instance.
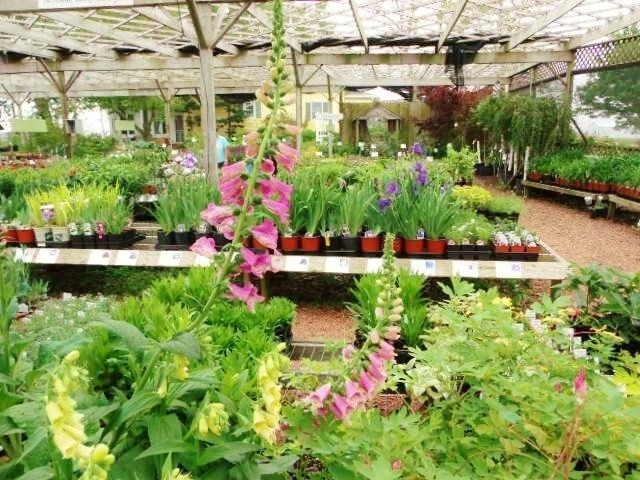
[360,140,463,239]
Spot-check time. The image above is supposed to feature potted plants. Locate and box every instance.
[138,152,361,252]
[446,184,542,262]
[0,109,66,168]
[265,296,297,351]
[343,268,486,401]
[528,149,640,202]
[1,156,149,249]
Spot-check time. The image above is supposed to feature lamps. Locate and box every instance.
[10,38,48,134]
[113,71,135,130]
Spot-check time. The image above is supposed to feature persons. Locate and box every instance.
[215,127,229,168]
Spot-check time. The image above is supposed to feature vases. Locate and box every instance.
[360,234,446,256]
